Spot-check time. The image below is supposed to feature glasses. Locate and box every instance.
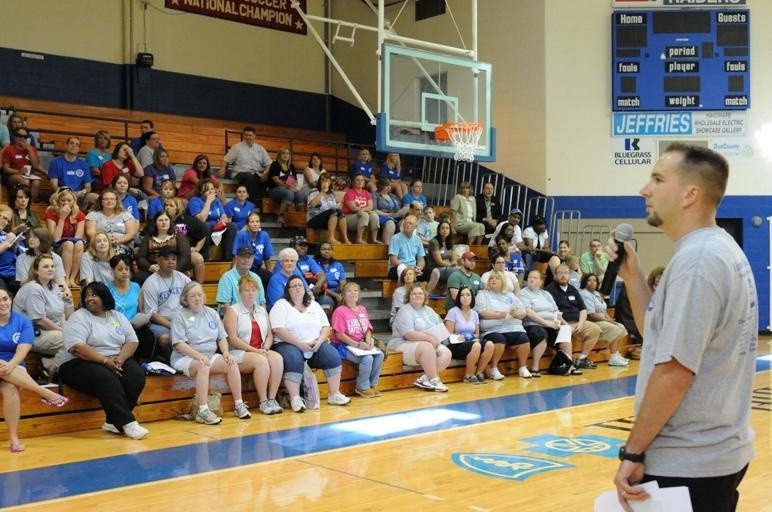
[557,271,570,275]
[0,214,10,225]
[57,188,72,193]
[413,185,424,188]
[103,198,117,201]
[14,131,28,140]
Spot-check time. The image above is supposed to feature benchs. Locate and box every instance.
[0,96,634,446]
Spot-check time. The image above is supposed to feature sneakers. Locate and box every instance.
[372,387,381,397]
[259,401,275,414]
[102,422,120,433]
[291,397,307,412]
[269,401,283,413]
[464,375,481,385]
[426,378,449,392]
[413,376,436,389]
[476,374,488,384]
[608,355,630,366]
[574,357,597,369]
[327,391,351,405]
[122,421,149,440]
[355,387,375,398]
[487,368,505,380]
[234,402,251,418]
[519,366,533,378]
[196,409,222,425]
[37,358,48,384]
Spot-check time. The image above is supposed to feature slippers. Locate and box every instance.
[41,393,68,407]
[571,368,583,373]
[532,370,541,377]
[11,442,24,452]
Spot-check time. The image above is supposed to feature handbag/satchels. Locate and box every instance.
[372,335,388,361]
[628,346,641,359]
[547,351,572,376]
[303,360,319,410]
[175,393,223,419]
[304,271,328,296]
[435,212,451,224]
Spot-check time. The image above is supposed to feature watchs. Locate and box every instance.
[616,446,643,461]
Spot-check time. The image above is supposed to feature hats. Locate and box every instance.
[510,208,522,216]
[236,246,253,256]
[159,246,177,256]
[131,310,154,331]
[290,236,309,245]
[462,252,478,259]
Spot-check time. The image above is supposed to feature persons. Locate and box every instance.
[1,115,384,451]
[374,152,664,393]
[603,142,759,512]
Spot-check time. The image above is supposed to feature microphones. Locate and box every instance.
[599,223,633,295]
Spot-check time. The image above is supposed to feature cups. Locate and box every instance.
[176,223,187,236]
[463,330,475,341]
[24,165,32,176]
[323,183,328,191]
